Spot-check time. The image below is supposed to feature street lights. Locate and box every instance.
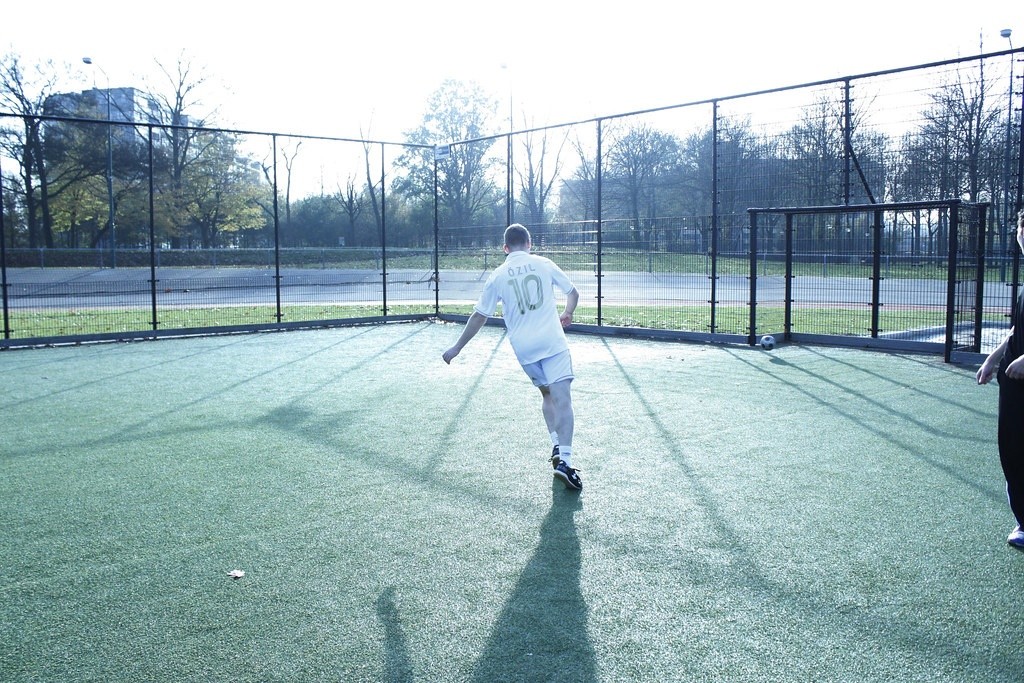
[999,28,1015,282]
[83,57,117,269]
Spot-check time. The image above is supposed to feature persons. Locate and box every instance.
[443,224,582,488]
[977,208,1024,546]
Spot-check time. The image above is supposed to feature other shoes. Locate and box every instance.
[1007,525,1024,547]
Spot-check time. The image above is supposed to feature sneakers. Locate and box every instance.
[548,445,560,469]
[553,460,582,490]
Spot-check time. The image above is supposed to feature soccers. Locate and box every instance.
[760,336,776,350]
